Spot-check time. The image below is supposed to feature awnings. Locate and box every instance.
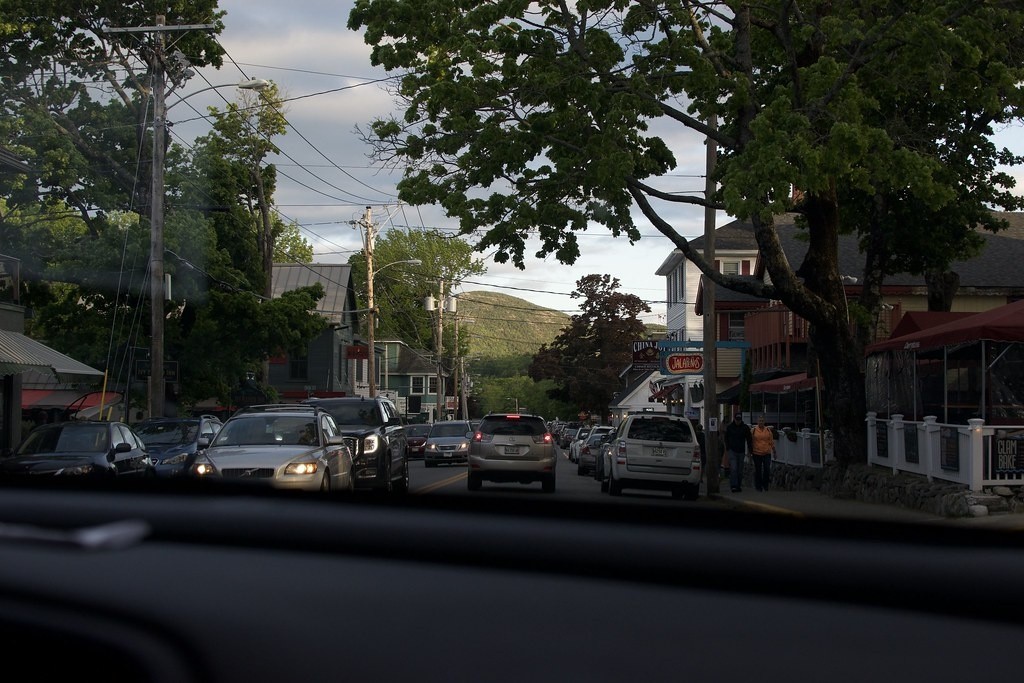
[0,330,105,385]
[749,373,816,394]
[22,389,86,409]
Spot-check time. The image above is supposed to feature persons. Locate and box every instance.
[692,419,707,483]
[749,415,777,491]
[724,413,753,492]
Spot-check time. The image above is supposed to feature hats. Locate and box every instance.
[734,413,743,420]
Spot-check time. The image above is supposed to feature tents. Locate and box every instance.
[869,298,1024,426]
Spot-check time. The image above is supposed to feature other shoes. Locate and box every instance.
[756,487,769,492]
[732,487,742,492]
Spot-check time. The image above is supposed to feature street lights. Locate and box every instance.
[151,79,269,419]
[368,259,424,398]
[436,317,476,420]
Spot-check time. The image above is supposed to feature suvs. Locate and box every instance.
[299,395,410,501]
[130,416,223,487]
[603,415,703,501]
[183,404,353,502]
[465,413,558,493]
[579,423,616,455]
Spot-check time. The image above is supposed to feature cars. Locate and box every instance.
[594,427,621,480]
[0,421,156,487]
[405,425,433,460]
[425,422,474,465]
[547,421,590,459]
[468,419,481,433]
